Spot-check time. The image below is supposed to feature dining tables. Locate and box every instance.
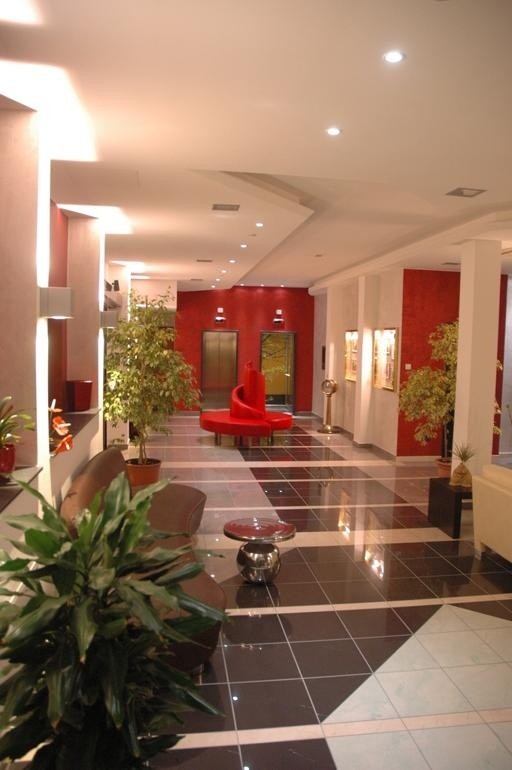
[0,393,38,485]
[446,446,472,486]
[106,287,204,486]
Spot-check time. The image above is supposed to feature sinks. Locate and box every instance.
[60,447,228,631]
[198,362,293,448]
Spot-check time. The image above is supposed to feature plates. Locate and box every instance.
[342,329,356,382]
[369,327,398,393]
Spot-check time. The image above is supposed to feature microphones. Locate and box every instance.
[225,519,298,590]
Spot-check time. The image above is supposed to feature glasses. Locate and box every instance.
[214,305,224,323]
[272,307,285,326]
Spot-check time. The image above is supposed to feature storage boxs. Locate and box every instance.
[428,476,473,537]
